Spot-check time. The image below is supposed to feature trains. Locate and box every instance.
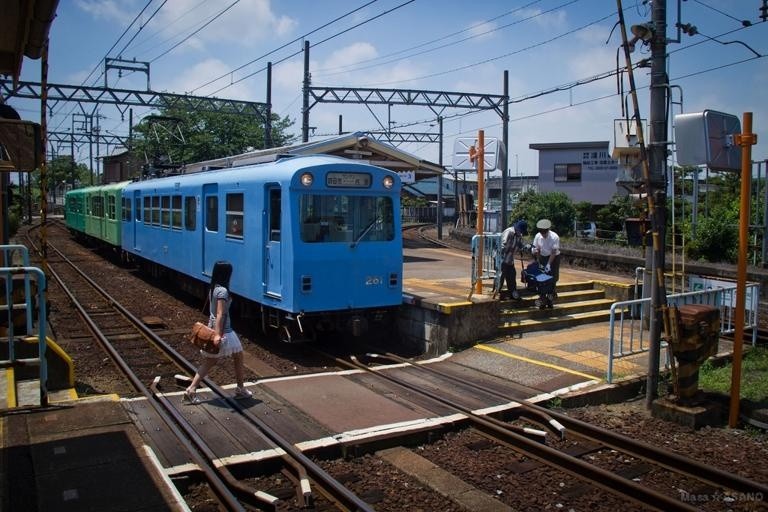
[65,155,403,343]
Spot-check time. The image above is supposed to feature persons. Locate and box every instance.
[181,259,254,406]
[532,218,561,310]
[491,221,533,301]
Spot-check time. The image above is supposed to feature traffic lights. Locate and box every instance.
[673,109,742,169]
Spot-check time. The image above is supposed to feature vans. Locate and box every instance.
[568,220,596,240]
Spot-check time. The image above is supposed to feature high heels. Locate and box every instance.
[233,386,253,400]
[181,388,201,405]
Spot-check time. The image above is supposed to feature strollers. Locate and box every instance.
[521,244,558,309]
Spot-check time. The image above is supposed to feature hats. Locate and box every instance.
[536,219,552,232]
[512,220,529,236]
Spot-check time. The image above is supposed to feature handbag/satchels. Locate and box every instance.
[189,321,221,355]
